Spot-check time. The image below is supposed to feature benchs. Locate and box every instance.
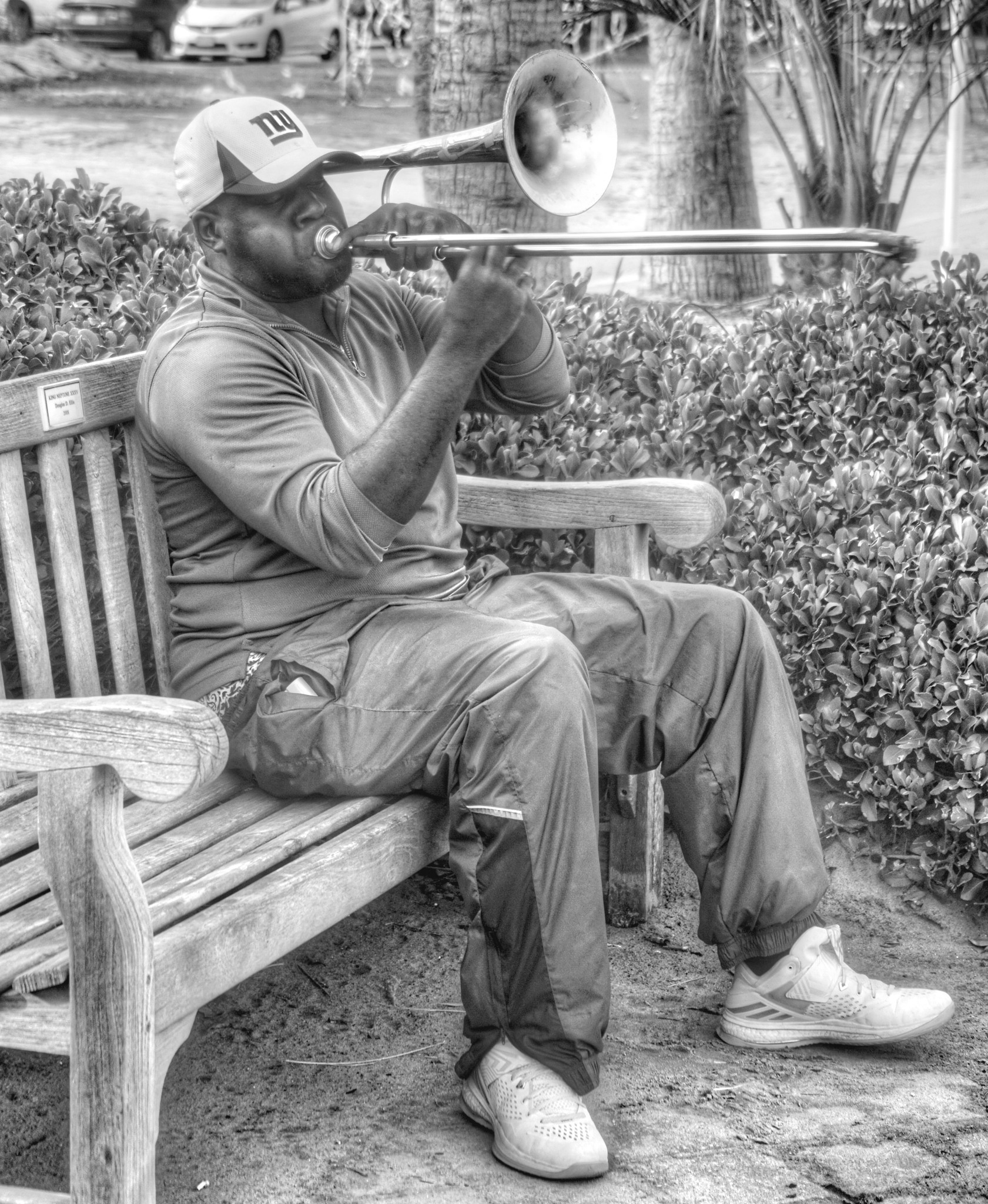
[0,350,726,1204]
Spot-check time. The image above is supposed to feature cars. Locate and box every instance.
[5,0,166,56]
[173,0,343,60]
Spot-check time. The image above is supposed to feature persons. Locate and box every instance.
[138,92,955,1177]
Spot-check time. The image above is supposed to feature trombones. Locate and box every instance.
[315,50,906,272]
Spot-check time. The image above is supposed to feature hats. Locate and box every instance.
[172,94,364,218]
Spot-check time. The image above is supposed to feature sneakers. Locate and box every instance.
[714,926,954,1052]
[459,1034,609,1180]
